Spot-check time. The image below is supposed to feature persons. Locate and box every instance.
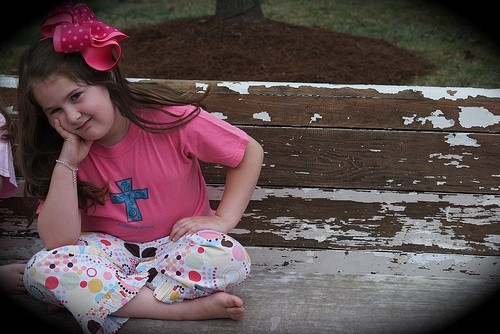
[0,89,27,296]
[16,3,264,334]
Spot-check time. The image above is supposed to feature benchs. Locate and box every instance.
[0,77,500,333]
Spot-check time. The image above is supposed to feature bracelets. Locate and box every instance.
[55,158,79,188]
